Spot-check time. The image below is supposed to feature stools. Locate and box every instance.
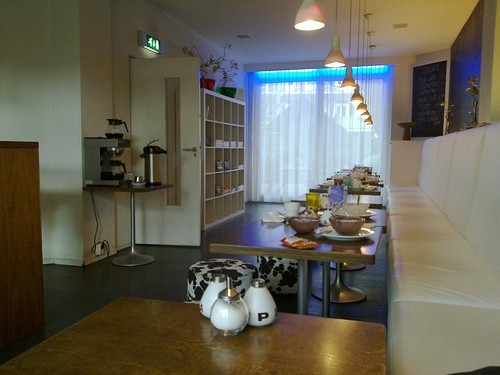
[257,257,298,294]
[186,259,258,299]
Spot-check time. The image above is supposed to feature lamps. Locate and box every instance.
[293,0,375,126]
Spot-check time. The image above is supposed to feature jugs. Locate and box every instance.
[109,159,127,180]
[139,140,168,187]
[104,118,129,139]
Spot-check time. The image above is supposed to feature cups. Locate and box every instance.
[135,176,142,183]
[284,201,301,217]
[334,178,343,186]
[306,192,320,215]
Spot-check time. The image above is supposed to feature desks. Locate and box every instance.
[0,297,386,375]
[84,181,174,266]
[210,182,386,314]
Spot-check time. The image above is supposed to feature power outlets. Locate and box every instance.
[96,244,105,255]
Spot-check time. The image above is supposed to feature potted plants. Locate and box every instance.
[182,42,216,90]
[214,40,238,97]
[464,76,478,128]
[441,102,455,136]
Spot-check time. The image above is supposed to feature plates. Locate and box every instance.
[331,210,377,220]
[321,228,375,241]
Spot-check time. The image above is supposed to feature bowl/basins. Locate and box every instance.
[288,217,321,235]
[329,215,365,236]
[361,185,378,192]
[343,195,370,216]
[349,172,376,187]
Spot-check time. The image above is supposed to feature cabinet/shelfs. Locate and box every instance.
[177,88,246,230]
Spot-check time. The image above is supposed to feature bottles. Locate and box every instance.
[242,278,278,327]
[209,277,250,337]
[199,274,227,320]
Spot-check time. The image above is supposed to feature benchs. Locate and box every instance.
[388,123,500,375]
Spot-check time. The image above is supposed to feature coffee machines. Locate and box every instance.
[84,136,131,187]
